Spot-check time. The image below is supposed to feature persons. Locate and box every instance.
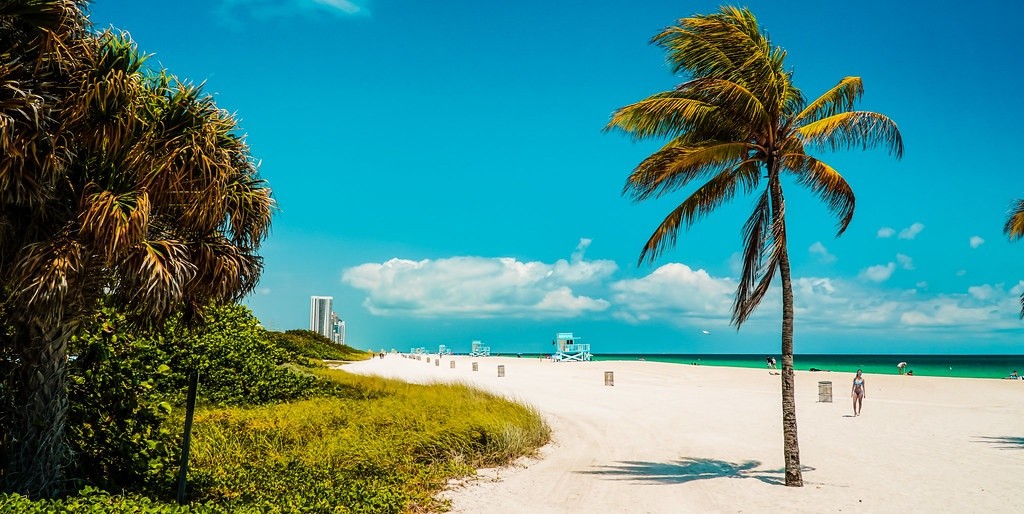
[1013,371,1018,377]
[851,369,865,417]
[766,356,777,369]
[949,364,952,371]
[897,361,912,376]
[380,353,382,359]
[635,356,638,360]
[373,353,375,358]
[641,355,644,359]
